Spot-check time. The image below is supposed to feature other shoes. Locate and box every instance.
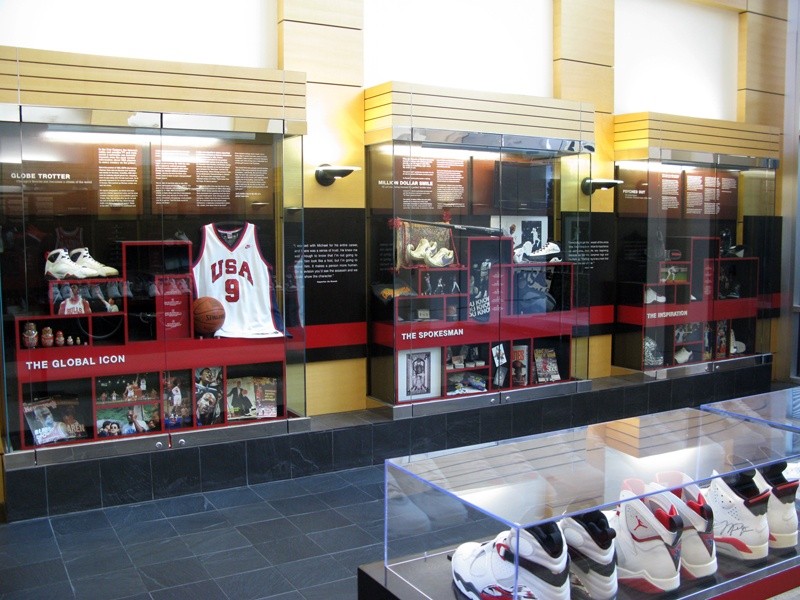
[719,287,730,298]
[727,284,741,298]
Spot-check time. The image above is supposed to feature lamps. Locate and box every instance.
[314,164,362,187]
[581,177,624,195]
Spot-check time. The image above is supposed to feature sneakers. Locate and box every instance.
[649,470,718,580]
[701,455,771,564]
[408,238,438,262]
[600,477,683,594]
[555,497,619,600]
[43,248,100,279]
[424,248,454,267]
[750,445,797,547]
[674,347,692,363]
[645,288,666,304]
[69,247,119,275]
[527,241,560,260]
[452,520,572,600]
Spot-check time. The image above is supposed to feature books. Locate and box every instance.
[511,345,529,387]
[22,397,89,446]
[533,349,561,381]
[226,376,277,420]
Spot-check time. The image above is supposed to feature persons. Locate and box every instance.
[665,266,677,283]
[542,350,549,372]
[30,405,68,441]
[227,381,242,417]
[97,366,220,436]
[96,291,119,312]
[240,389,253,416]
[475,256,492,299]
[422,272,461,294]
[58,285,91,315]
[62,403,81,436]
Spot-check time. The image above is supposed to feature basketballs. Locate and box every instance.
[192,297,225,334]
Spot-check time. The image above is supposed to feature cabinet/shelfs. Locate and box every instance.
[7,240,287,452]
[363,235,575,408]
[610,236,760,371]
